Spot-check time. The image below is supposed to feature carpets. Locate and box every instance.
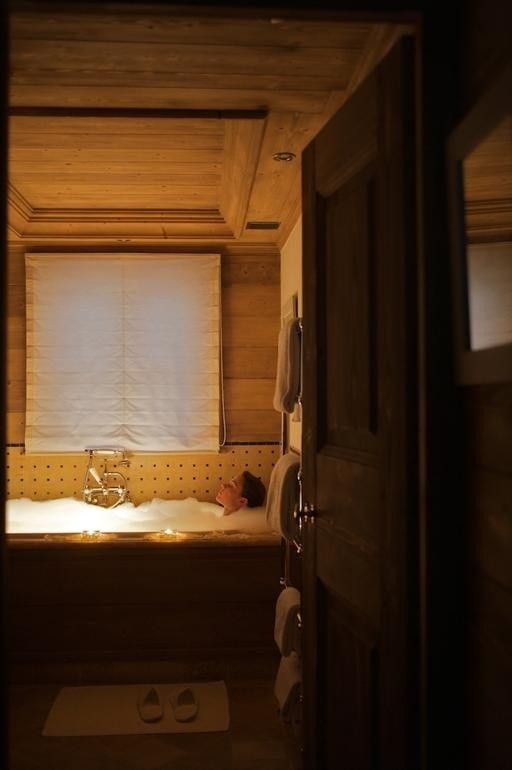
[41,683,230,738]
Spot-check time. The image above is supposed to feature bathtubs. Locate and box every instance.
[4,530,281,652]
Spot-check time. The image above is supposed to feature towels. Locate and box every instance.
[273,656,301,736]
[272,587,301,657]
[264,453,299,542]
[271,318,300,416]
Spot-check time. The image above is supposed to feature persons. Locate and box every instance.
[215,470,266,516]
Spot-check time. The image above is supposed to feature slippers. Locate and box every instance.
[171,687,198,721]
[139,686,163,721]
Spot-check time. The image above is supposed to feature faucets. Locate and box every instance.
[79,447,132,510]
